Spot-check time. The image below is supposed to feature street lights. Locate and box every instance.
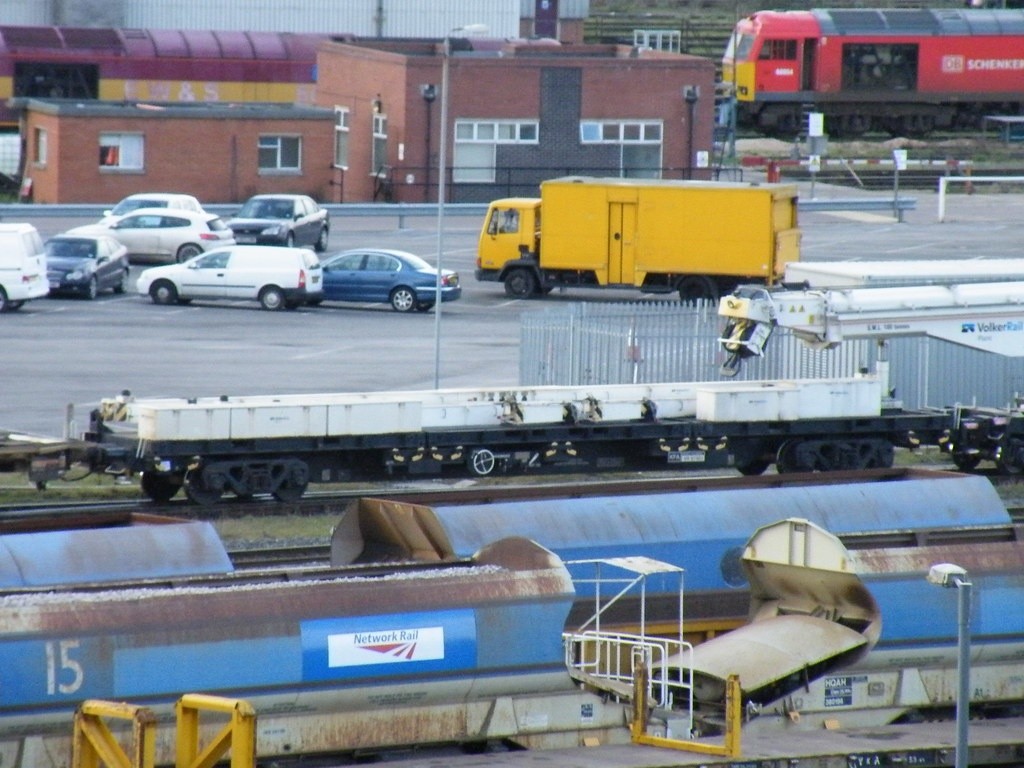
[431,23,489,387]
[925,562,974,768]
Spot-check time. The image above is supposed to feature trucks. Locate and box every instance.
[475,174,804,311]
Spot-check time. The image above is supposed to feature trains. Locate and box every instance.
[720,6,1024,140]
[0,358,1024,768]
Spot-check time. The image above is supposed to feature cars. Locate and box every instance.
[41,235,131,300]
[63,206,235,268]
[96,194,206,226]
[321,248,461,312]
[224,192,331,253]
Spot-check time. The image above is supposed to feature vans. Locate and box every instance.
[136,245,326,315]
[0,224,50,313]
